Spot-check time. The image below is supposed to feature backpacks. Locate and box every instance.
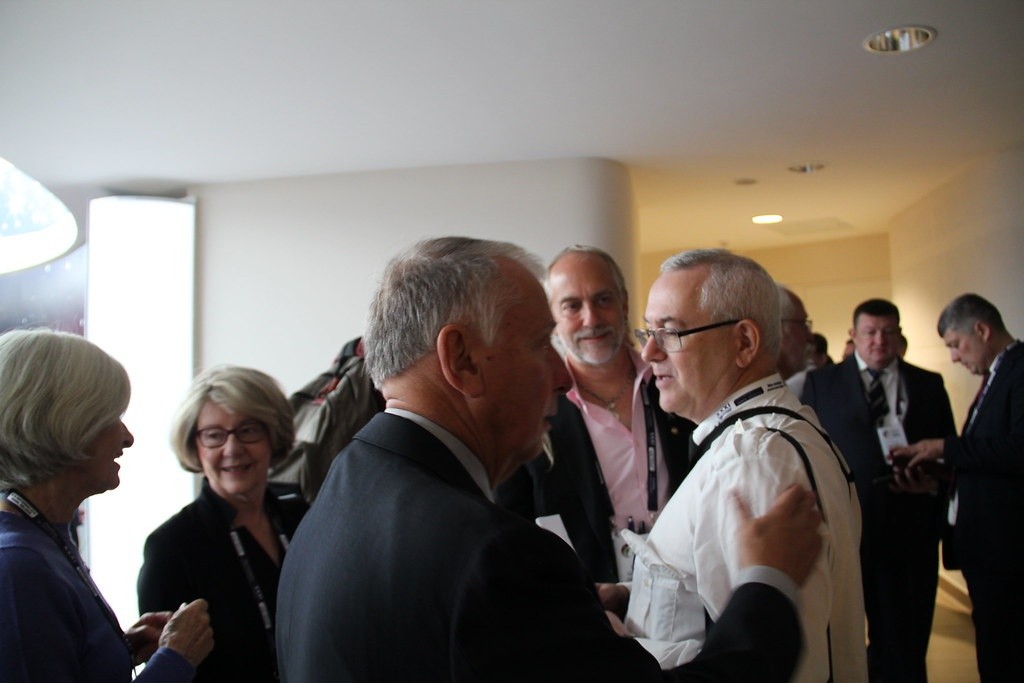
[267,337,374,507]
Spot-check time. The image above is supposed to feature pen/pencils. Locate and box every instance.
[638,520,645,534]
[627,515,635,532]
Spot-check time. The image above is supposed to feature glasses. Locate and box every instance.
[196,422,268,447]
[633,319,742,352]
[778,320,813,333]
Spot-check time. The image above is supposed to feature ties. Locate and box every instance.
[866,366,889,421]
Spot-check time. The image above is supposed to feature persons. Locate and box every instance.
[801,297,959,683]
[885,292,1023,683]
[528,244,694,614]
[134,335,385,683]
[0,325,215,683]
[602,247,868,683]
[777,284,910,406]
[273,235,826,683]
